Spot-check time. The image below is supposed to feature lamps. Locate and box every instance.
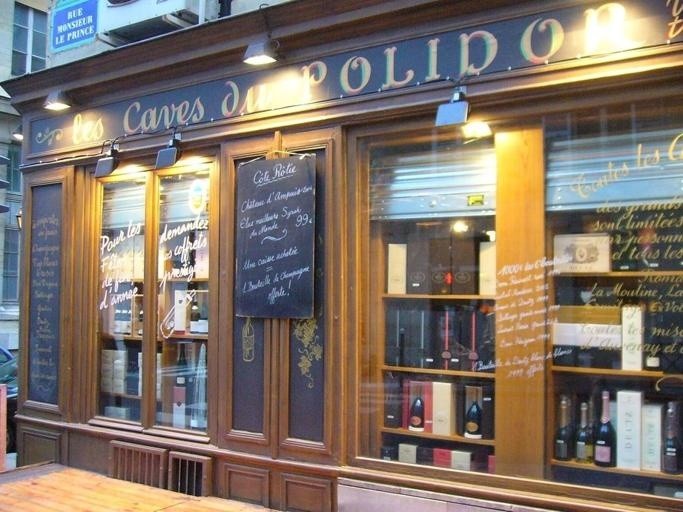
[148,126,183,170]
[90,126,120,183]
[240,9,284,68]
[431,77,473,129]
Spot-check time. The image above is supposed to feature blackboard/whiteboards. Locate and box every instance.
[235,154,317,318]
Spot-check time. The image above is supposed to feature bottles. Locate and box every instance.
[192,297,208,322]
[465,388,481,436]
[661,401,683,477]
[592,389,618,469]
[161,288,197,337]
[408,383,424,429]
[171,341,190,386]
[555,388,596,464]
[112,299,144,336]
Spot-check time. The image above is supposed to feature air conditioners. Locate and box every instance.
[99,0,222,47]
